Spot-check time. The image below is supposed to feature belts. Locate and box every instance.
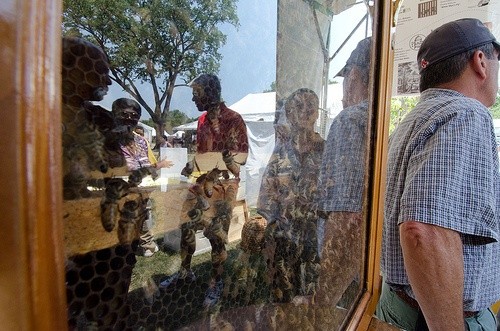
[396,288,478,318]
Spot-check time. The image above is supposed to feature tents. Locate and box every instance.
[138,122,155,145]
[172,81,344,204]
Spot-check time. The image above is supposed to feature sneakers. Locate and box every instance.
[143,245,158,257]
[202,279,223,309]
[158,269,196,292]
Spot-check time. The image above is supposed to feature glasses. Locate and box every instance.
[117,111,138,119]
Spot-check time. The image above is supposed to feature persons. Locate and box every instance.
[377,17,500,331]
[59,32,372,331]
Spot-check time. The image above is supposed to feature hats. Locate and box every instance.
[333,36,372,79]
[417,18,500,74]
